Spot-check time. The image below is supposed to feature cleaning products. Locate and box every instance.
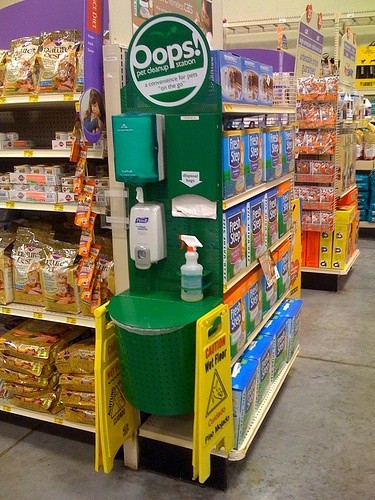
[178,234,205,303]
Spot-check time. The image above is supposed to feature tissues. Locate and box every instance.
[112,112,165,204]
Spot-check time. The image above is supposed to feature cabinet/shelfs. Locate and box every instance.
[0,45,375,470]
